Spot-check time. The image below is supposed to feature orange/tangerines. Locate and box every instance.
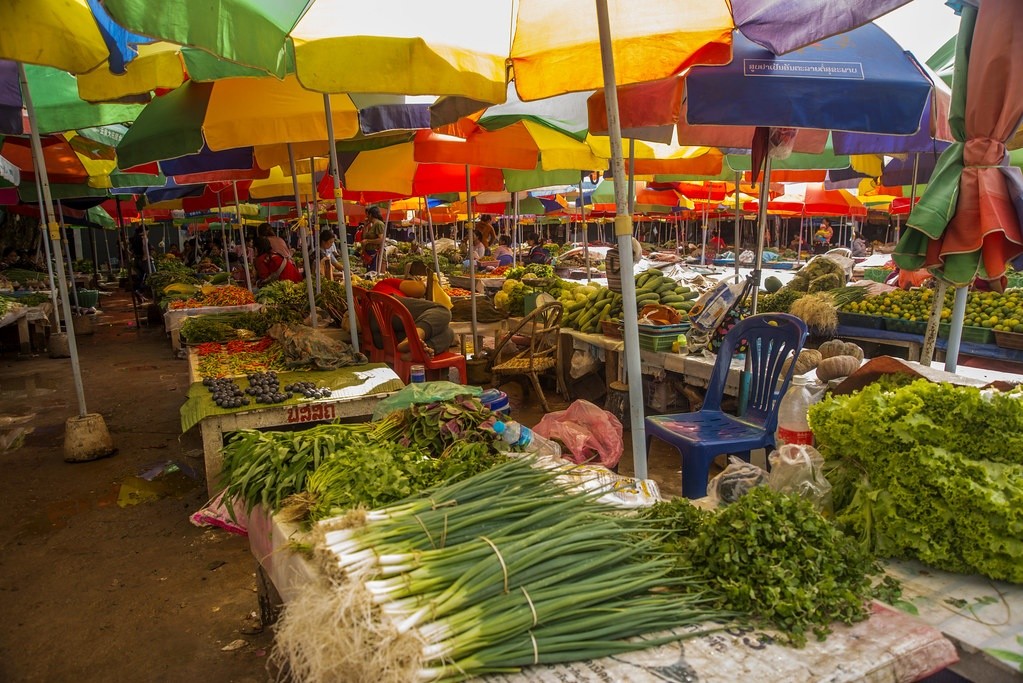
[842,286,1023,335]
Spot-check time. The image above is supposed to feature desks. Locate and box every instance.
[1,274,93,360]
[167,277,1023,683]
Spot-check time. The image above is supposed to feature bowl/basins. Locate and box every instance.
[476,259,502,268]
[69,290,98,308]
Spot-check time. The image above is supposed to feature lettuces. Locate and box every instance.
[807,372,1023,588]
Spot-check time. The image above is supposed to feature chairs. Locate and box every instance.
[645,311,808,500]
[477,248,550,272]
[351,284,385,364]
[363,288,468,388]
[483,301,571,414]
[826,247,852,259]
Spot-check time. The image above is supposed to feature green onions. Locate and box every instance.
[0,296,23,316]
[274,435,752,683]
[790,284,868,329]
[4,269,51,283]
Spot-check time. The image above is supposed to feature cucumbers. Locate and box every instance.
[559,269,698,333]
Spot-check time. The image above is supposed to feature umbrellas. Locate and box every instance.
[0,0,1023,490]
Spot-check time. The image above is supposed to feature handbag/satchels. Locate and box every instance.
[360,250,371,265]
[689,274,747,331]
[605,252,622,292]
[532,251,551,265]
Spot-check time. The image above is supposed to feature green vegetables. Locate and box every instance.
[331,241,482,276]
[179,311,270,343]
[208,397,526,535]
[618,484,911,646]
[48,257,94,274]
[254,271,348,327]
[504,244,575,315]
[755,288,799,315]
[18,291,49,307]
[117,252,204,287]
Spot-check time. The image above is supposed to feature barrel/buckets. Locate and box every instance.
[567,366,607,402]
[480,387,510,416]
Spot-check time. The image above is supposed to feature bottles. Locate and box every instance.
[411,365,426,384]
[494,421,564,461]
[677,334,690,356]
[777,375,815,449]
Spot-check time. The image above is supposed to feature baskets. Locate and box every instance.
[618,320,691,353]
[599,320,625,337]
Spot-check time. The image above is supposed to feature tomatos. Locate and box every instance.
[493,274,602,322]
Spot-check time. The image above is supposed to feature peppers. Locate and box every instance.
[192,337,311,379]
[490,265,509,276]
[443,287,472,296]
[170,285,257,309]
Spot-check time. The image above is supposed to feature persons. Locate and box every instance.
[369,278,454,362]
[234,237,253,263]
[354,206,386,279]
[709,231,727,250]
[790,232,810,251]
[492,235,514,263]
[252,238,304,288]
[168,243,180,255]
[853,231,867,257]
[309,230,343,276]
[518,232,550,264]
[0,246,42,271]
[132,224,154,303]
[258,223,295,266]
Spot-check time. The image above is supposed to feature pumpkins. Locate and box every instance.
[781,339,864,381]
